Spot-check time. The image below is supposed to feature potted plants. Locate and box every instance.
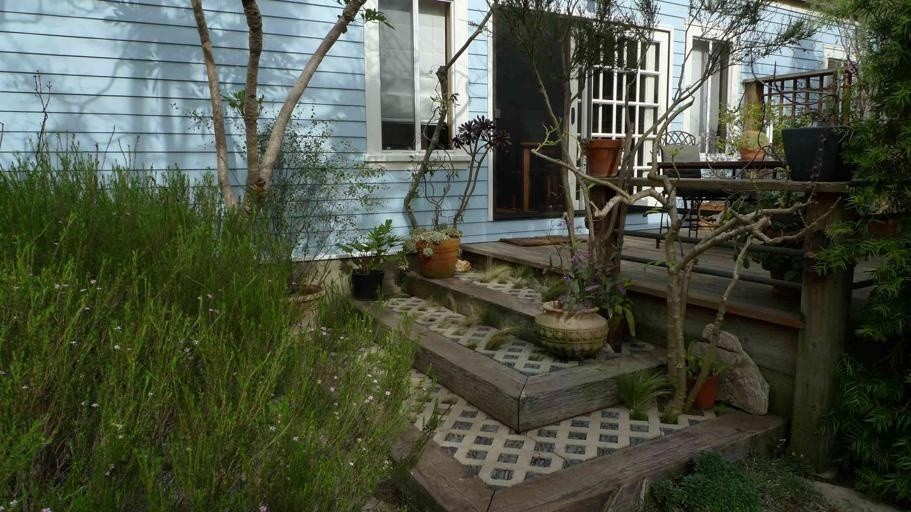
[336,219,463,301]
[171,95,391,338]
[738,130,770,159]
[686,338,729,410]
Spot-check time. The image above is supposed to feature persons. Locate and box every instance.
[512,89,562,211]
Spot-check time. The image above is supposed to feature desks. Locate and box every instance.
[647,159,791,239]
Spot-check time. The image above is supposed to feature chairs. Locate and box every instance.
[656,131,704,248]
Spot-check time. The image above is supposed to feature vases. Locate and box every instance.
[535,300,610,360]
[583,139,626,179]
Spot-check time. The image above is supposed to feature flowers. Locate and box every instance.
[547,217,637,339]
